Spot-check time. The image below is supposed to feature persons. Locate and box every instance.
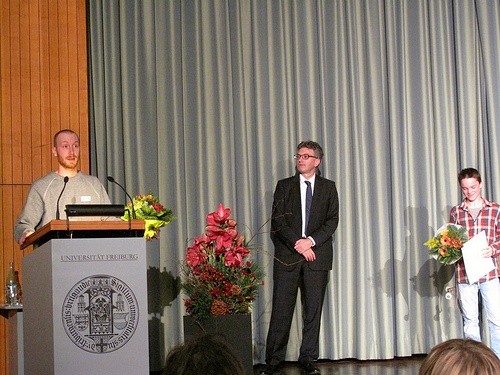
[264,141,339,375]
[15,129,124,246]
[449,168,500,359]
[419,339,500,375]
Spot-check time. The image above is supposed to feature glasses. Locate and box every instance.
[293,154,319,160]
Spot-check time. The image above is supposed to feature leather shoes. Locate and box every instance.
[299,360,321,375]
[259,360,285,375]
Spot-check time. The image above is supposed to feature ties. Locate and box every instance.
[304,181,312,235]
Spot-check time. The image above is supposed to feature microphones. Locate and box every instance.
[107,176,136,219]
[56,176,69,220]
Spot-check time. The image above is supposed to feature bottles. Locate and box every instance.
[6,262,18,306]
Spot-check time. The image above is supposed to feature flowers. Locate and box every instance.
[177,198,307,317]
[425,224,468,266]
[122,191,177,241]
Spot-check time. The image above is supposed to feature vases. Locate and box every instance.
[184,311,253,375]
